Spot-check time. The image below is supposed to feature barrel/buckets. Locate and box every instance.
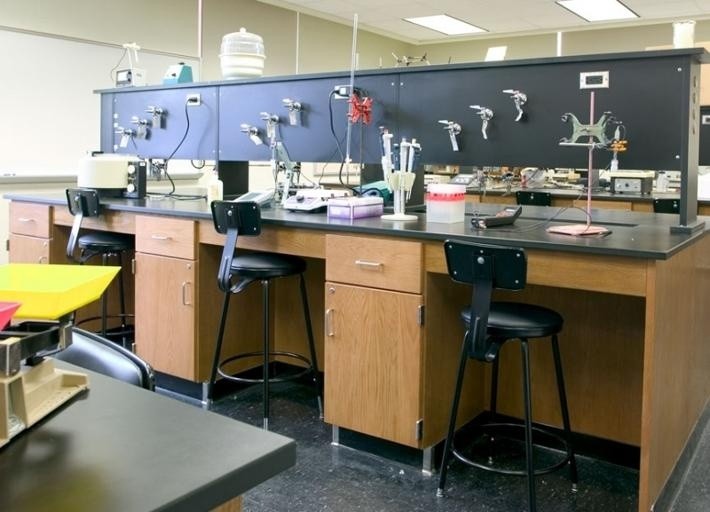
[672,19,696,49]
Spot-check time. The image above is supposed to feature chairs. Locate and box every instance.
[46,326,156,391]
[207,200,324,430]
[435,238,577,511]
[65,188,135,348]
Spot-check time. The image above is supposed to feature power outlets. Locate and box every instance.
[579,70,609,90]
[186,94,200,106]
[334,85,350,99]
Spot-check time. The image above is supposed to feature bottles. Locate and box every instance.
[206,171,225,207]
[656,171,670,193]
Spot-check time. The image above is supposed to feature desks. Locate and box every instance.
[465,189,710,217]
[0,359,294,512]
[4,188,710,512]
